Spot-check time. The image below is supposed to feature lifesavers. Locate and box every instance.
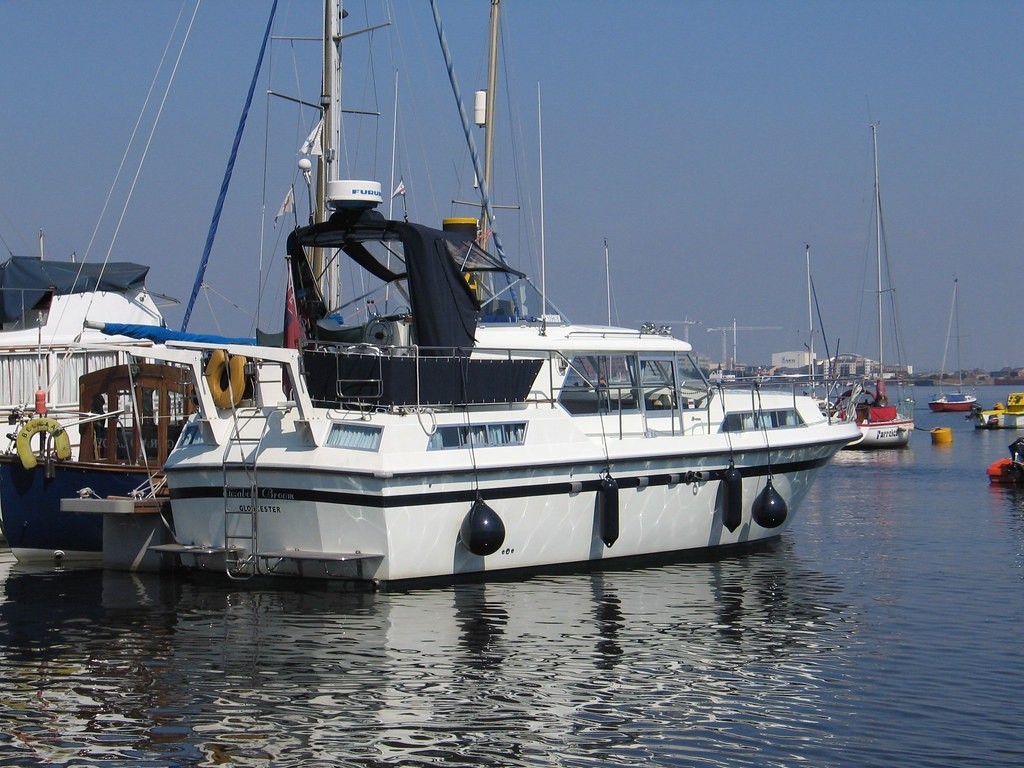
[15,417,72,471]
[206,349,248,410]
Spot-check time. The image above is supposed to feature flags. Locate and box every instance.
[392,181,406,199]
[274,190,294,229]
[295,118,323,155]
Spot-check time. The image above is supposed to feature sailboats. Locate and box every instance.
[818,121,916,446]
[928,277,978,412]
[1,3,862,591]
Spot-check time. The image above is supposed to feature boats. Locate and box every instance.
[985,437,1024,484]
[965,392,1024,428]
